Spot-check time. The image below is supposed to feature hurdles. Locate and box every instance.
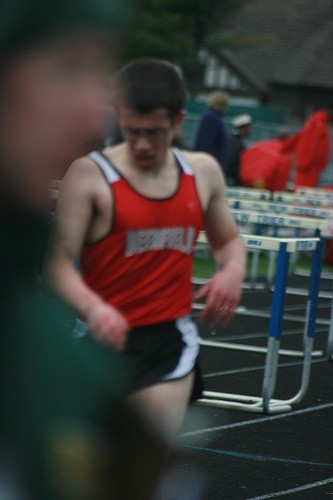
[42,175,332,415]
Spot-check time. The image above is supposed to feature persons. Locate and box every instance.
[227,114,252,183]
[0,0,206,500]
[196,90,230,176]
[240,132,293,190]
[292,108,333,188]
[48,61,246,435]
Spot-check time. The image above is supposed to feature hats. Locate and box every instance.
[232,112,255,127]
